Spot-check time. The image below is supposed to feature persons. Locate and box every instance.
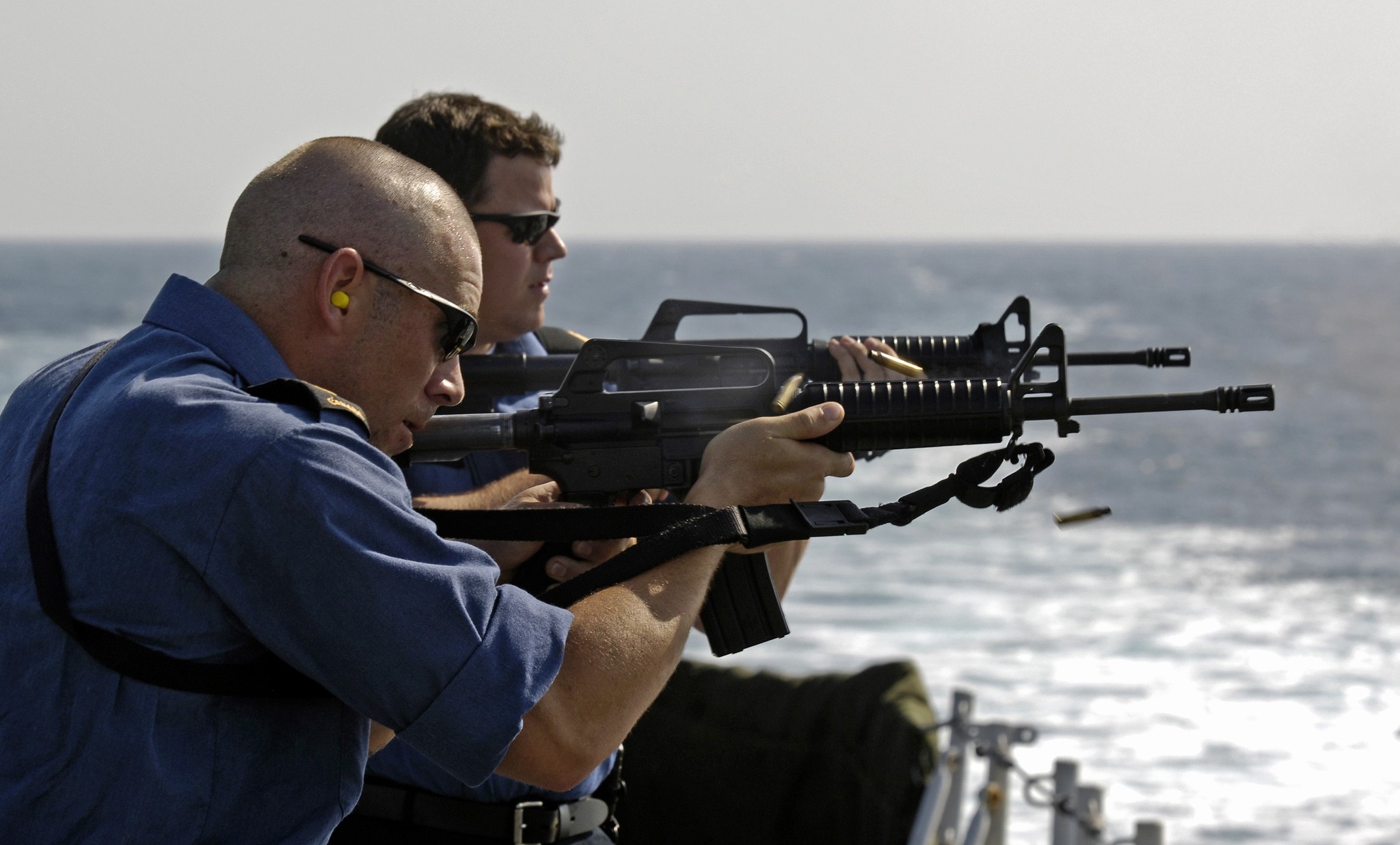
[331,91,911,845]
[0,133,857,845]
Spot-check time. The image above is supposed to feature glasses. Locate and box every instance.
[297,229,480,366]
[469,198,562,246]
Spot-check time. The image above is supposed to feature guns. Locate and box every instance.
[448,295,1194,487]
[392,335,1280,659]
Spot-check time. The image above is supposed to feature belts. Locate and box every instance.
[352,780,630,845]
